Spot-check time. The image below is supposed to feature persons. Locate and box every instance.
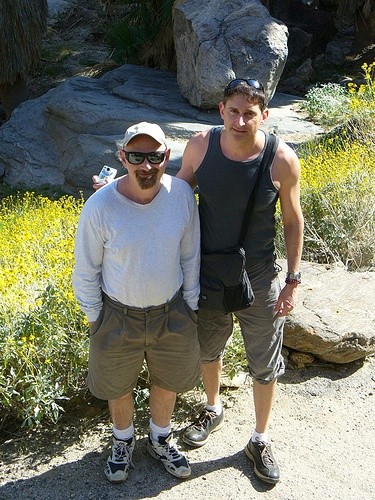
[72,122,200,481]
[93,78,304,482]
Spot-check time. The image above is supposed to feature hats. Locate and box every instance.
[122,122,165,147]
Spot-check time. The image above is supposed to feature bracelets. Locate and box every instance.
[285,279,298,287]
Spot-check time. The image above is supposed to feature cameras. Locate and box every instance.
[96,166,117,185]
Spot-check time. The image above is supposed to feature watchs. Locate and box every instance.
[286,271,302,284]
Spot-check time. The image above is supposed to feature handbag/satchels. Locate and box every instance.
[198,244,254,311]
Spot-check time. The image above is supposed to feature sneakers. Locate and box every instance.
[146,433,191,478]
[104,435,135,482]
[245,437,280,483]
[182,409,224,446]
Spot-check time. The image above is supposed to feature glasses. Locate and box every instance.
[225,79,264,93]
[123,149,166,164]
